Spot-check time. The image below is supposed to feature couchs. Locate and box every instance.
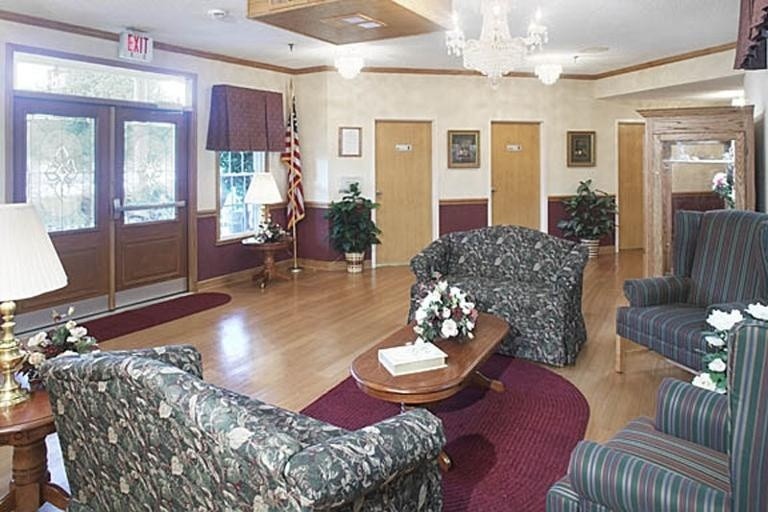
[544,321,768,511]
[42,344,451,511]
[406,224,588,369]
[613,208,763,375]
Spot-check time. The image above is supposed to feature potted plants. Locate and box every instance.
[322,178,387,275]
[556,177,625,259]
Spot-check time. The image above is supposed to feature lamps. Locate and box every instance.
[243,173,282,237]
[443,1,548,85]
[0,203,68,410]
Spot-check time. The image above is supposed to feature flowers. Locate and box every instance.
[412,280,476,342]
[689,302,766,394]
[16,307,96,383]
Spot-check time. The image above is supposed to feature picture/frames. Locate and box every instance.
[565,130,595,167]
[338,126,361,156]
[447,131,480,167]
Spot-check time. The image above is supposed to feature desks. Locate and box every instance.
[0,382,76,511]
[241,237,294,289]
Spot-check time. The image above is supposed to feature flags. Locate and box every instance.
[280,95,307,229]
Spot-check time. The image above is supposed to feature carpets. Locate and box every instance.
[79,293,231,343]
[299,356,593,511]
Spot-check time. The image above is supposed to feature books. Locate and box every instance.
[378,342,449,378]
